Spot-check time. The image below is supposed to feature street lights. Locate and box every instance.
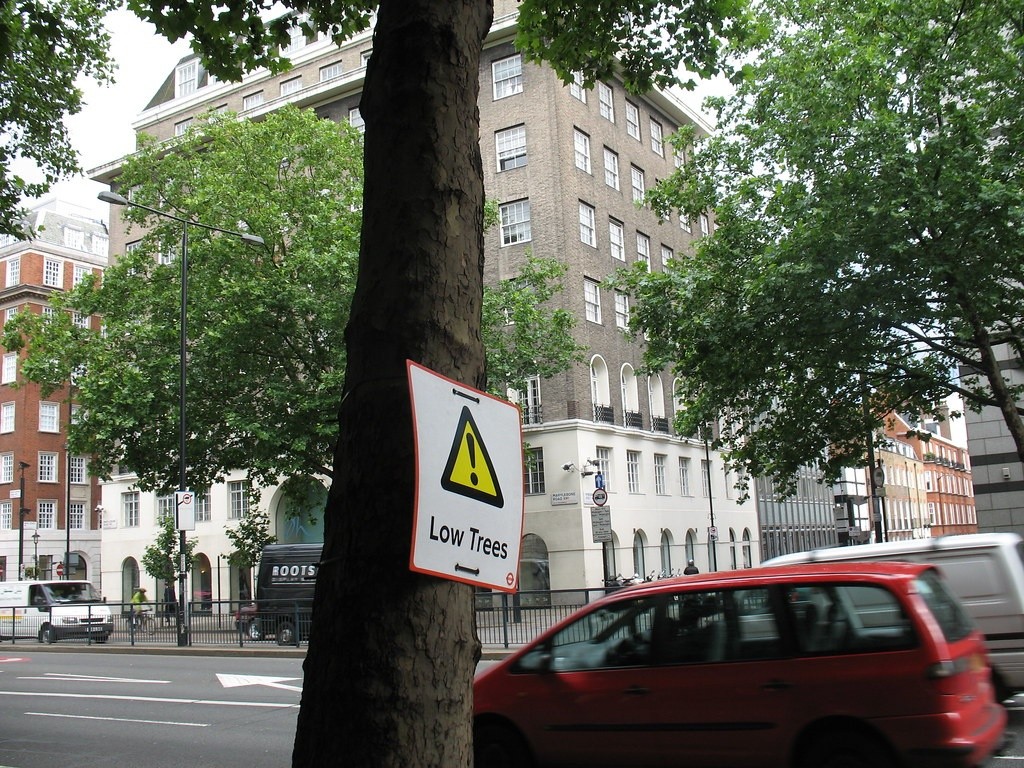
[94,189,266,650]
[17,461,31,581]
[30,529,40,581]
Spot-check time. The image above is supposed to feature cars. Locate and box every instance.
[235,603,267,641]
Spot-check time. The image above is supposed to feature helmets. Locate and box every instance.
[688,560,694,566]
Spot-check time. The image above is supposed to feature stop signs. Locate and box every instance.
[56,564,65,576]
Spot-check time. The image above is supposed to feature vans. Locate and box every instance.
[470,563,1010,768]
[0,580,115,644]
[684,535,1024,704]
[250,544,322,646]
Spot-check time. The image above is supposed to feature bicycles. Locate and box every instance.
[125,609,156,636]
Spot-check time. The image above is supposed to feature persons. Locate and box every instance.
[684,560,699,574]
[164,582,176,622]
[128,588,152,632]
